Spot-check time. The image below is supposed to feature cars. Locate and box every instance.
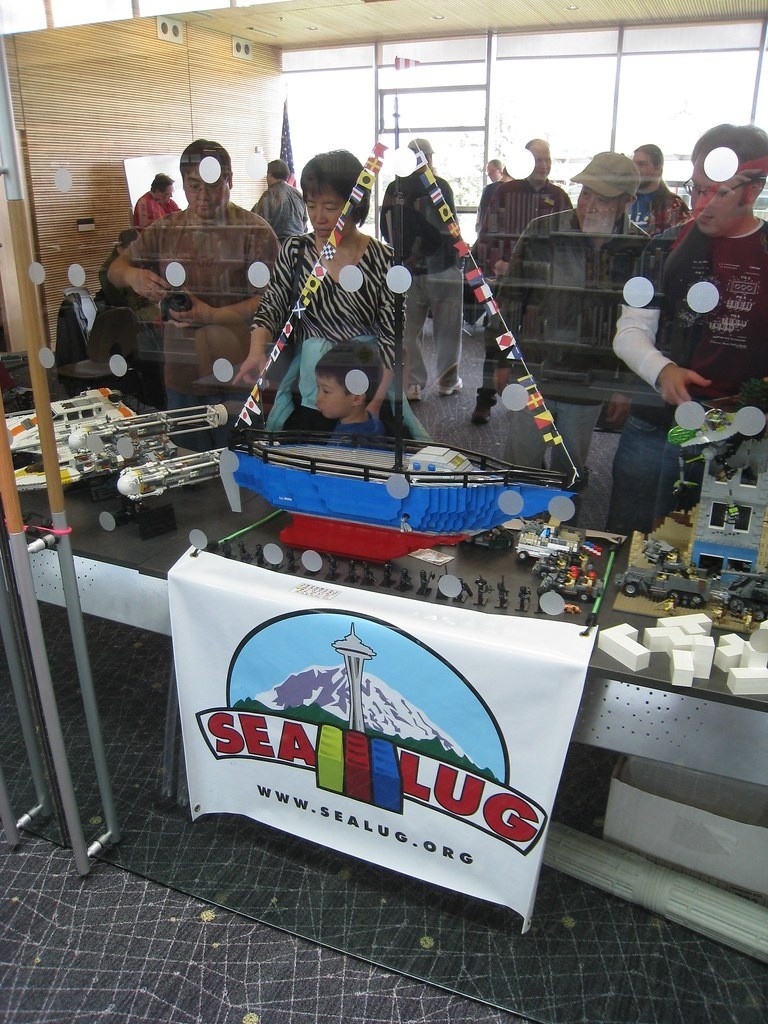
[464,524,515,552]
[532,547,603,603]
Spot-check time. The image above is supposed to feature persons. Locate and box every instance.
[97,229,164,349]
[252,159,309,245]
[471,138,572,423]
[379,138,464,400]
[316,340,387,448]
[667,548,698,579]
[134,174,180,236]
[107,139,280,451]
[665,596,753,631]
[607,123,768,540]
[231,151,408,433]
[494,152,650,523]
[628,144,692,236]
[222,540,531,611]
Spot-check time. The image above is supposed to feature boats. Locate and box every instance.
[3,386,176,491]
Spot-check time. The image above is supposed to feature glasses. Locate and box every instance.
[683,176,766,203]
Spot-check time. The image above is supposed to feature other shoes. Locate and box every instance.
[473,403,491,424]
[592,412,607,432]
[614,417,629,434]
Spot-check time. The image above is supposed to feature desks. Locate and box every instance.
[0,437,767,896]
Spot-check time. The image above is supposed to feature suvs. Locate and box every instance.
[616,537,768,624]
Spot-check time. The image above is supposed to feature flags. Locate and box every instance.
[281,108,297,187]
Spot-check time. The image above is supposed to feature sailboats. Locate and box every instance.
[222,91,582,563]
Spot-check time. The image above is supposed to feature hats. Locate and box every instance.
[408,139,435,156]
[570,151,642,198]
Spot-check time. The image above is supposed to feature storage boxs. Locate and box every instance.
[602,755,766,893]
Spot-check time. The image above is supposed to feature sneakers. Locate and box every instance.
[406,385,422,400]
[438,378,463,396]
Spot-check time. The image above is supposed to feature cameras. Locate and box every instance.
[161,290,193,322]
